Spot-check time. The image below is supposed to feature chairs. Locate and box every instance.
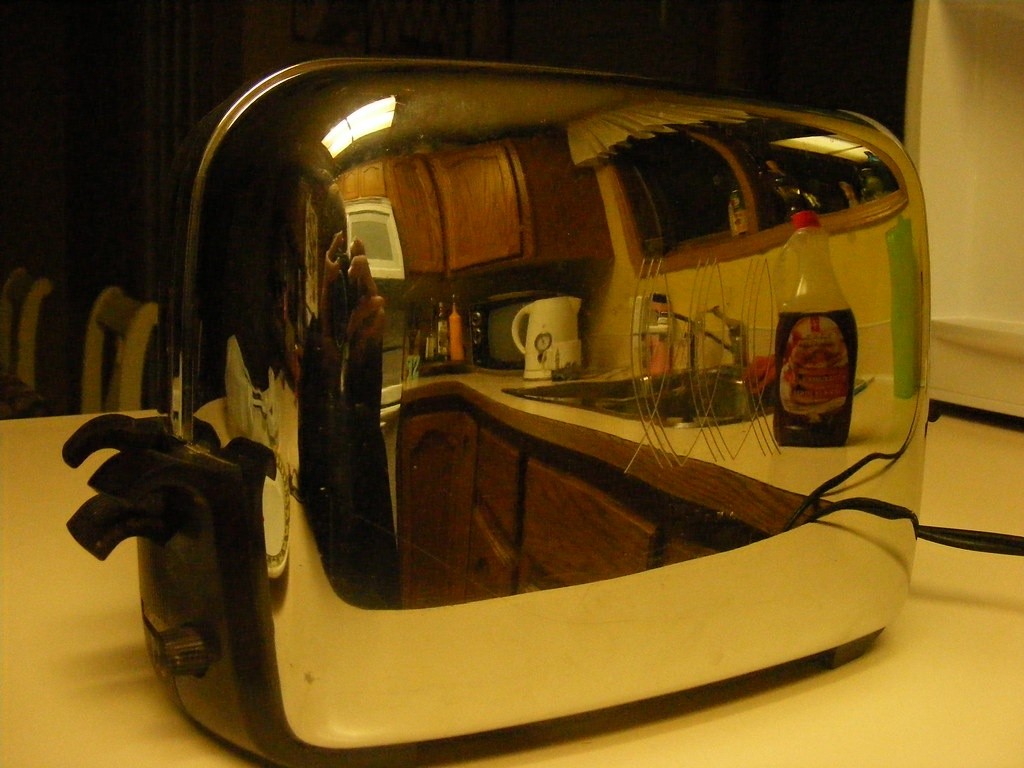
[80,287,161,414]
[0,268,52,419]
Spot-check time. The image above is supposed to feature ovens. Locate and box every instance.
[343,198,405,280]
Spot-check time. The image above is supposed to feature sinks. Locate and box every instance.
[501,364,724,404]
[592,374,778,428]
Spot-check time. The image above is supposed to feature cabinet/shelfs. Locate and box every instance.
[527,457,723,600]
[397,412,476,607]
[466,428,516,603]
[336,133,615,284]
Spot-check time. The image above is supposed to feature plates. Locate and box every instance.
[262,454,291,578]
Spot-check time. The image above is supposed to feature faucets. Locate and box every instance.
[663,305,749,375]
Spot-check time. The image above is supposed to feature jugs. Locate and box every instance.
[511,296,581,378]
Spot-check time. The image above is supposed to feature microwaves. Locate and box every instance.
[471,291,551,370]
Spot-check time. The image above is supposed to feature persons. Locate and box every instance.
[295,170,401,609]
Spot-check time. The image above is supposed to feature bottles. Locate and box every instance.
[449,303,465,360]
[437,302,448,360]
[424,323,437,361]
[771,213,858,445]
[727,188,748,237]
[649,294,672,375]
[743,146,786,228]
